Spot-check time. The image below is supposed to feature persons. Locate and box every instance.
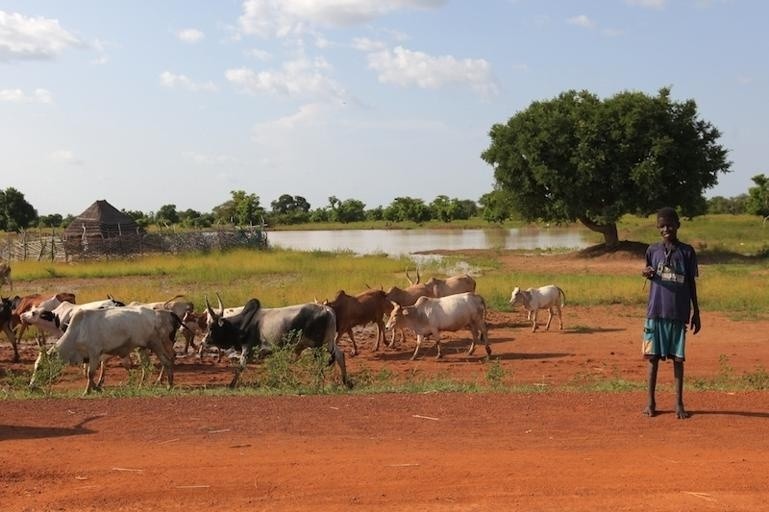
[641,207,701,418]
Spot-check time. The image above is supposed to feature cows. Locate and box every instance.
[509,284,565,332]
[128,296,245,363]
[203,292,354,392]
[365,266,476,346]
[26,305,181,395]
[313,288,389,354]
[385,292,492,361]
[0,291,128,361]
